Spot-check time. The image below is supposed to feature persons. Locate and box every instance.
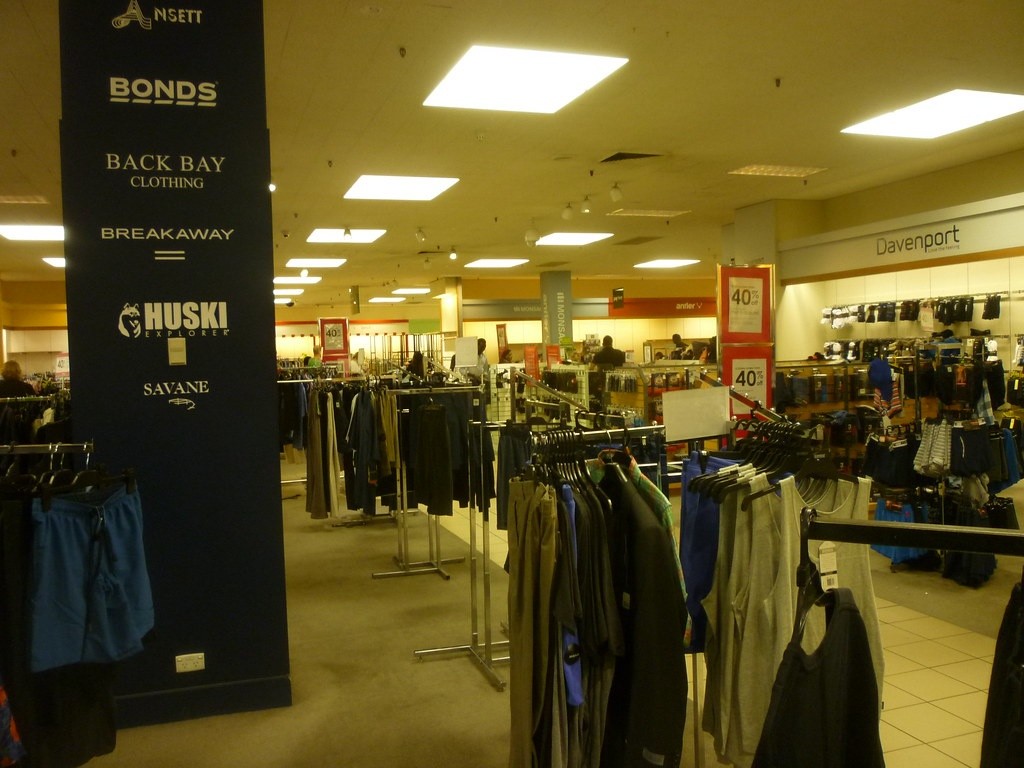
[402,351,424,381]
[808,352,823,360]
[308,344,323,367]
[0,359,37,397]
[499,350,513,363]
[588,335,625,371]
[460,338,488,386]
[654,334,716,365]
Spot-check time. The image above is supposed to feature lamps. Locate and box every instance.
[562,180,623,220]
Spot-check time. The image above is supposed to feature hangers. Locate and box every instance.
[0,395,138,502]
[499,339,1024,647]
[275,357,479,396]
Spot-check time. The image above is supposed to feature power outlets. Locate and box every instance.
[175,653,205,673]
